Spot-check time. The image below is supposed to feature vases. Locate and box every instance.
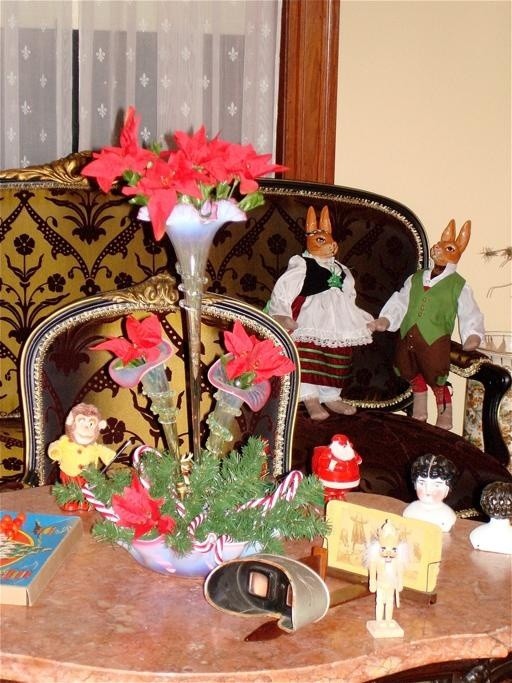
[94,197,279,574]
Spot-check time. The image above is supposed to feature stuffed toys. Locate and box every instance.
[371,217,484,431]
[268,205,376,420]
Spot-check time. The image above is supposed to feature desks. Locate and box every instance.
[0,477,512,683]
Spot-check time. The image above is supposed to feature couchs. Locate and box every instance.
[1,149,512,522]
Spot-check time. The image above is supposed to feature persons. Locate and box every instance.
[403,452,512,555]
[311,434,363,503]
[364,519,405,639]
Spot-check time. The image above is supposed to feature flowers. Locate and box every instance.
[48,105,336,557]
